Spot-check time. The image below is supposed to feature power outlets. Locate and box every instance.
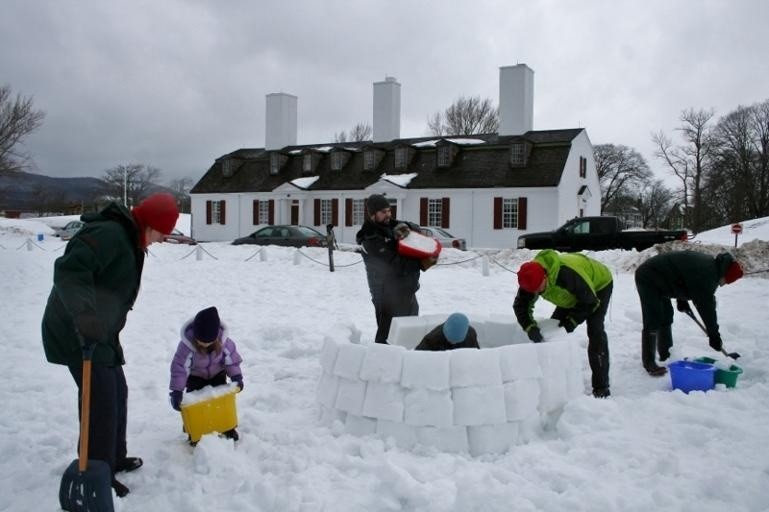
[512,214,690,253]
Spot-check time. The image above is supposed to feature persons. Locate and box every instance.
[169,306,244,446]
[41,192,179,497]
[414,312,480,351]
[512,249,613,398]
[635,249,743,376]
[356,193,422,344]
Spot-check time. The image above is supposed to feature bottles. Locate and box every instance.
[724,258,744,284]
[517,263,546,292]
[366,193,390,216]
[443,313,469,343]
[194,306,219,343]
[132,192,179,249]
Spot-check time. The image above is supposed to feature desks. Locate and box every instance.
[120,160,131,208]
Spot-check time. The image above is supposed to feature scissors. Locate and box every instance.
[686,309,740,360]
[59,343,115,512]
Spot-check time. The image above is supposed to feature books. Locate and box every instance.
[694,356,743,388]
[181,388,239,443]
[668,361,717,393]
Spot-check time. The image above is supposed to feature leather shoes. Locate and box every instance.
[169,391,182,412]
[677,300,690,312]
[709,335,722,351]
[231,375,243,392]
[559,314,577,332]
[528,327,543,342]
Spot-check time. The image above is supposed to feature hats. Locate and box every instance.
[50,219,84,240]
[225,222,328,248]
[159,228,198,246]
[416,225,469,252]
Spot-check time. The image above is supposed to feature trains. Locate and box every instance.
[730,223,743,236]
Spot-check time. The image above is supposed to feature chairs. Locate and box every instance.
[592,387,610,398]
[225,429,239,442]
[117,457,142,472]
[648,366,667,375]
[111,480,129,497]
